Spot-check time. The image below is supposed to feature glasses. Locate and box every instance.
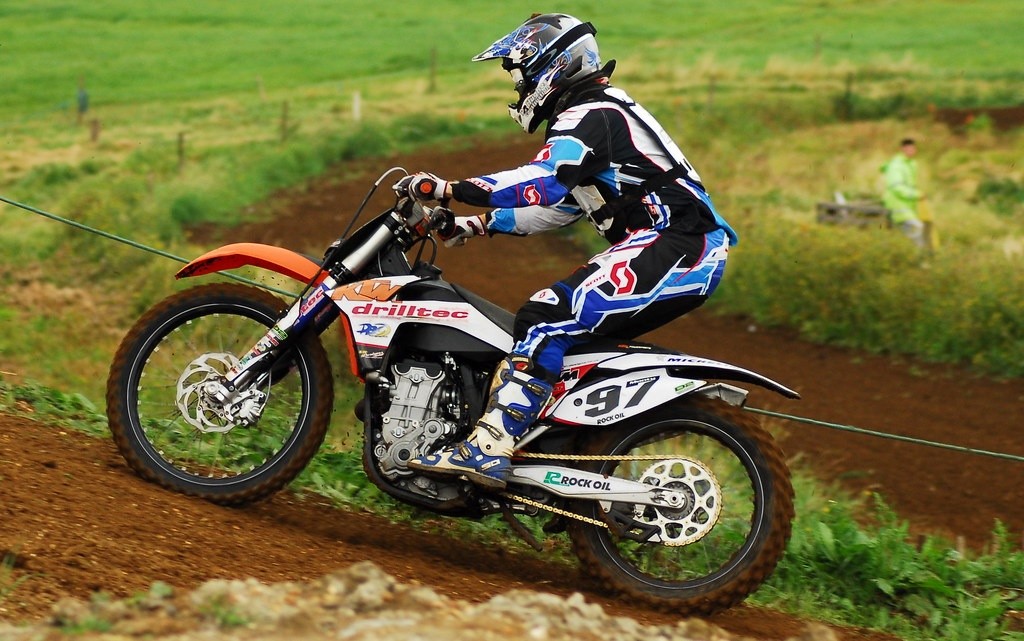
[504,62,528,99]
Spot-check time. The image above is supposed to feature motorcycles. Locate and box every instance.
[106,166,802,618]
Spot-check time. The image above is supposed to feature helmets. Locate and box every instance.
[471,12,602,134]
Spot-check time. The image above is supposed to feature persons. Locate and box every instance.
[880,139,926,247]
[394,12,738,487]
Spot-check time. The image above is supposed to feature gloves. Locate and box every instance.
[438,215,486,248]
[392,171,447,201]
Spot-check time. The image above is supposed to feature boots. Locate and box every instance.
[408,354,554,488]
